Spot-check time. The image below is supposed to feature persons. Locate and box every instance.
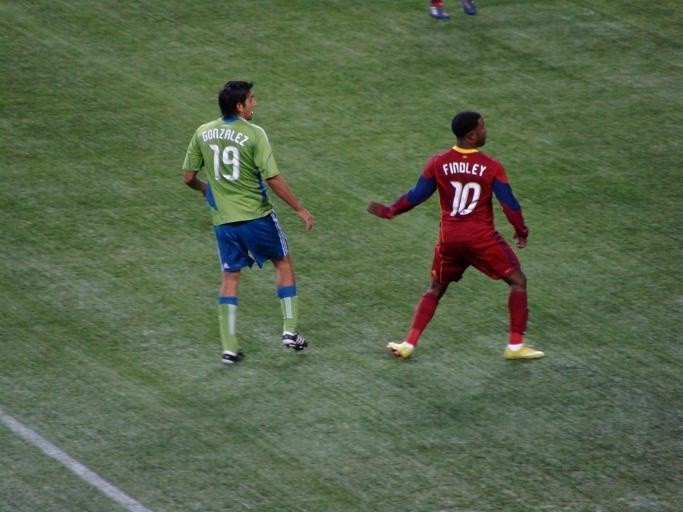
[428,0,477,19]
[366,111,547,361]
[182,80,313,365]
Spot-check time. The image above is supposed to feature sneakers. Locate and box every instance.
[282,331,308,351]
[504,344,546,360]
[463,0,476,15]
[386,342,415,359]
[430,6,450,19]
[221,349,245,365]
